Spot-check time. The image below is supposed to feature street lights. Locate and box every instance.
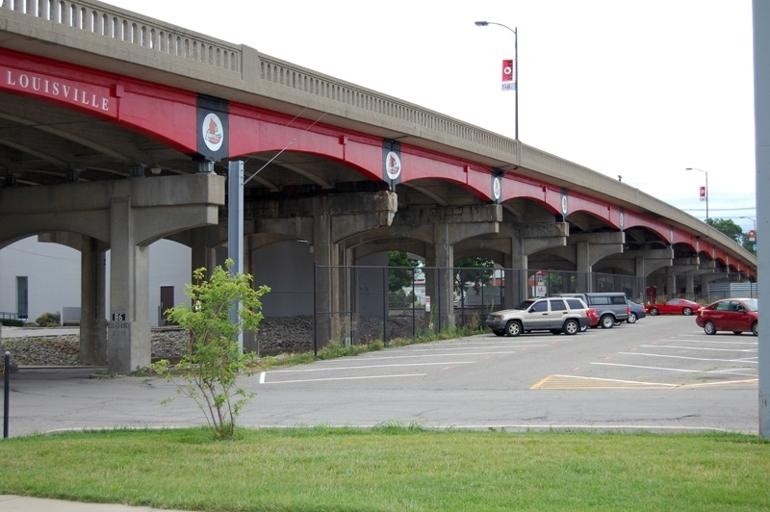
[740,215,756,259]
[687,167,710,223]
[477,20,519,142]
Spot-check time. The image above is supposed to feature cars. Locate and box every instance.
[627,299,646,324]
[696,297,758,335]
[644,299,700,315]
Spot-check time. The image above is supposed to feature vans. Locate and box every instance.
[555,292,631,329]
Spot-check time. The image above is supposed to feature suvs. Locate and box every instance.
[487,297,599,336]
